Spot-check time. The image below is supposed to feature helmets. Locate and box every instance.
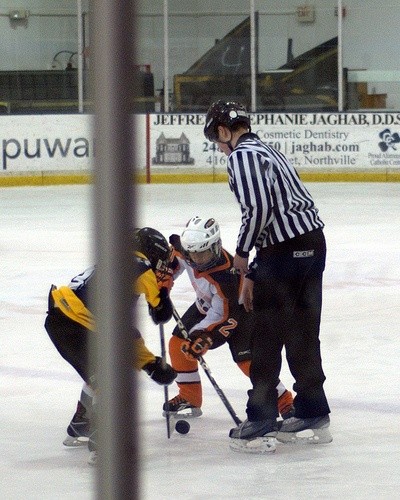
[180,215,222,270]
[204,99,251,142]
[131,226,173,282]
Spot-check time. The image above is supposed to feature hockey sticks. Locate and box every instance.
[157,322,176,438]
[172,308,283,432]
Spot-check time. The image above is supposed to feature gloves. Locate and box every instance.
[147,293,173,325]
[180,330,214,361]
[142,356,178,386]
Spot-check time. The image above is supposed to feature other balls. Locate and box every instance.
[174,420,190,435]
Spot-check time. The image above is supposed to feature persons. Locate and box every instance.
[45,228,177,466]
[157,217,294,423]
[204,99,334,454]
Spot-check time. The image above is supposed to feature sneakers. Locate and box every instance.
[281,403,295,420]
[229,414,278,454]
[63,401,92,446]
[86,426,100,465]
[162,396,203,419]
[275,413,333,444]
